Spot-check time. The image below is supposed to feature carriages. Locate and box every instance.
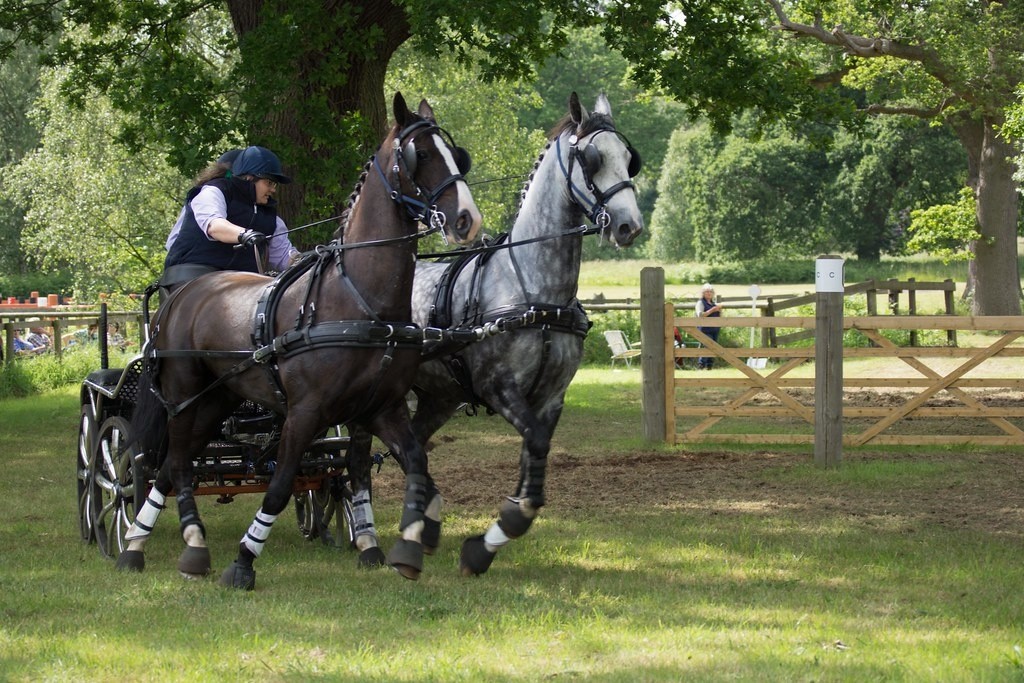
[75,89,646,591]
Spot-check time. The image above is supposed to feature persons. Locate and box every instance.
[0,318,136,362]
[695,283,722,371]
[163,145,302,296]
[674,327,686,369]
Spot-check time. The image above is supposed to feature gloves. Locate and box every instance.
[237,230,267,250]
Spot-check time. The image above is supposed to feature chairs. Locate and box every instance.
[604,330,642,373]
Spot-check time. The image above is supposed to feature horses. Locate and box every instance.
[114,91,485,591]
[346,91,646,578]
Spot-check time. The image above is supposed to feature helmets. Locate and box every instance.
[232,145,292,184]
[218,150,245,167]
